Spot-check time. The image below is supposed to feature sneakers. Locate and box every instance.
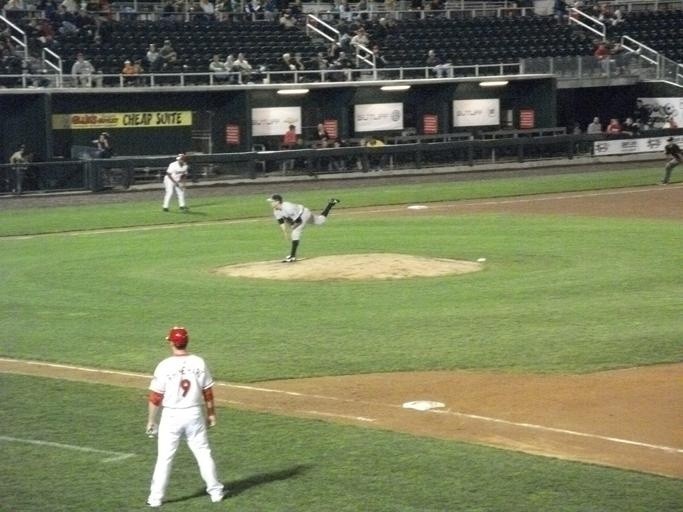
[144,500,162,508]
[326,197,341,205]
[179,206,189,212]
[209,489,230,503]
[280,254,297,263]
[162,208,169,213]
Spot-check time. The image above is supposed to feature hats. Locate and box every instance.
[667,137,674,142]
[266,194,283,202]
[175,153,187,161]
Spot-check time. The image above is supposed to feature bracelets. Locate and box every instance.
[206,407,213,417]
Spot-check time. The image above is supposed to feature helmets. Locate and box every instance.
[164,325,190,349]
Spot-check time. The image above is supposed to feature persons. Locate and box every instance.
[0,31,99,88]
[0,0,298,31]
[266,194,340,263]
[160,153,190,212]
[554,0,643,70]
[8,143,35,194]
[122,39,178,73]
[90,131,115,193]
[425,1,445,18]
[424,49,454,78]
[318,0,395,81]
[660,136,681,185]
[407,0,425,20]
[143,326,231,510]
[622,117,635,132]
[585,116,601,157]
[358,134,388,171]
[605,118,623,133]
[281,124,297,175]
[312,123,329,173]
[210,53,306,81]
